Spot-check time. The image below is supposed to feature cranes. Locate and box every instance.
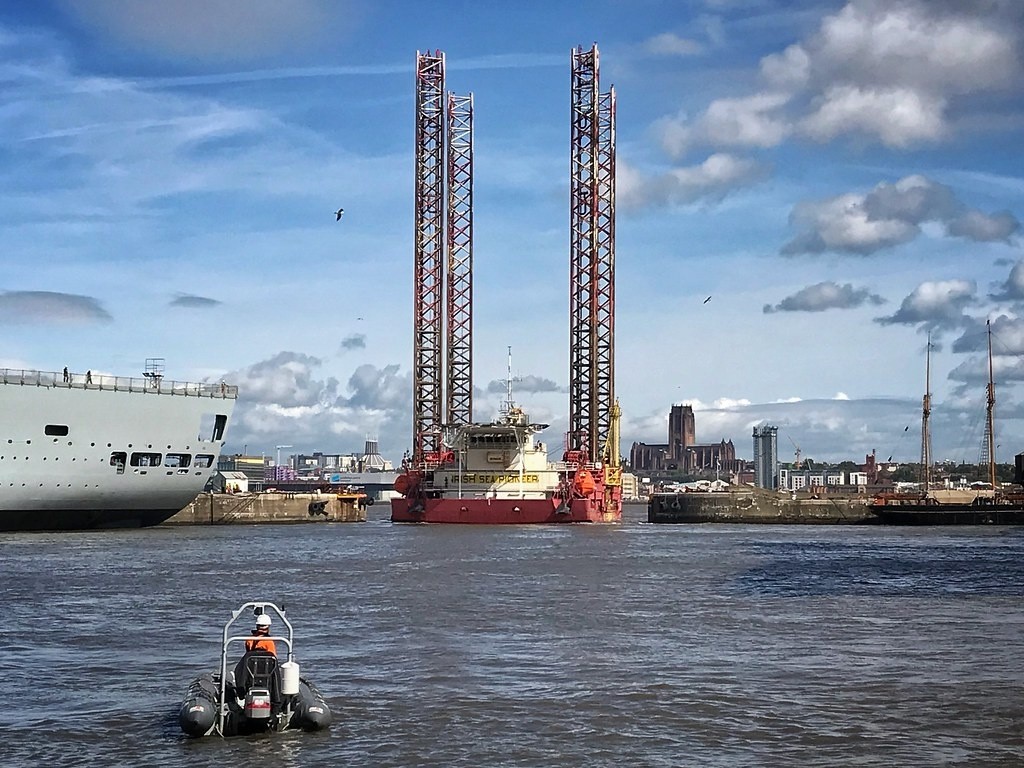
[786,433,801,470]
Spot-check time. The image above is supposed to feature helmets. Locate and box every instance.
[255,615,272,625]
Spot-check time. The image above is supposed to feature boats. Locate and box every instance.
[0,358,240,533]
[177,603,332,737]
[388,345,621,529]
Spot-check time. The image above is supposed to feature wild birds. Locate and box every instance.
[703,295,712,305]
[333,209,344,222]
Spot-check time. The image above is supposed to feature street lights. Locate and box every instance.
[274,444,294,467]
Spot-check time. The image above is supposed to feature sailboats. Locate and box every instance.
[868,320,1022,526]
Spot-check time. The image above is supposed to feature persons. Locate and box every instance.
[243,614,278,662]
